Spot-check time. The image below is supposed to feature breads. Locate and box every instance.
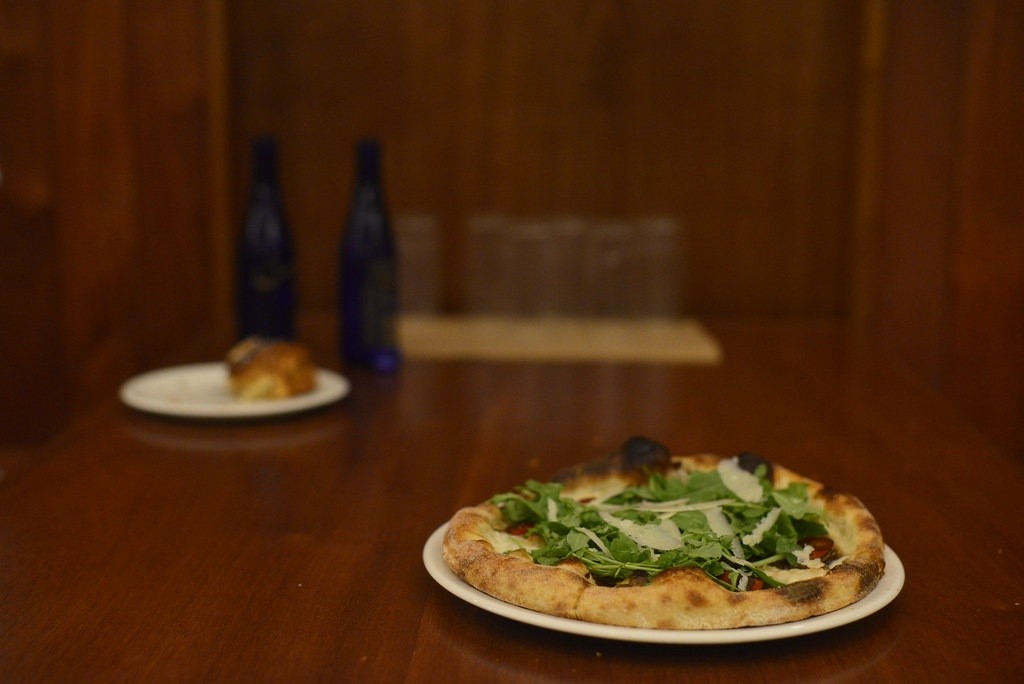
[226,337,318,399]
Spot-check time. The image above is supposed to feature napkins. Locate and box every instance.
[396,313,722,367]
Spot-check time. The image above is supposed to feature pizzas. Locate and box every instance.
[443,435,887,630]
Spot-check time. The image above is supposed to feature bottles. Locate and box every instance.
[234,134,296,344]
[335,137,401,374]
[119,362,349,416]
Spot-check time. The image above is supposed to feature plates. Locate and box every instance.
[422,518,905,643]
